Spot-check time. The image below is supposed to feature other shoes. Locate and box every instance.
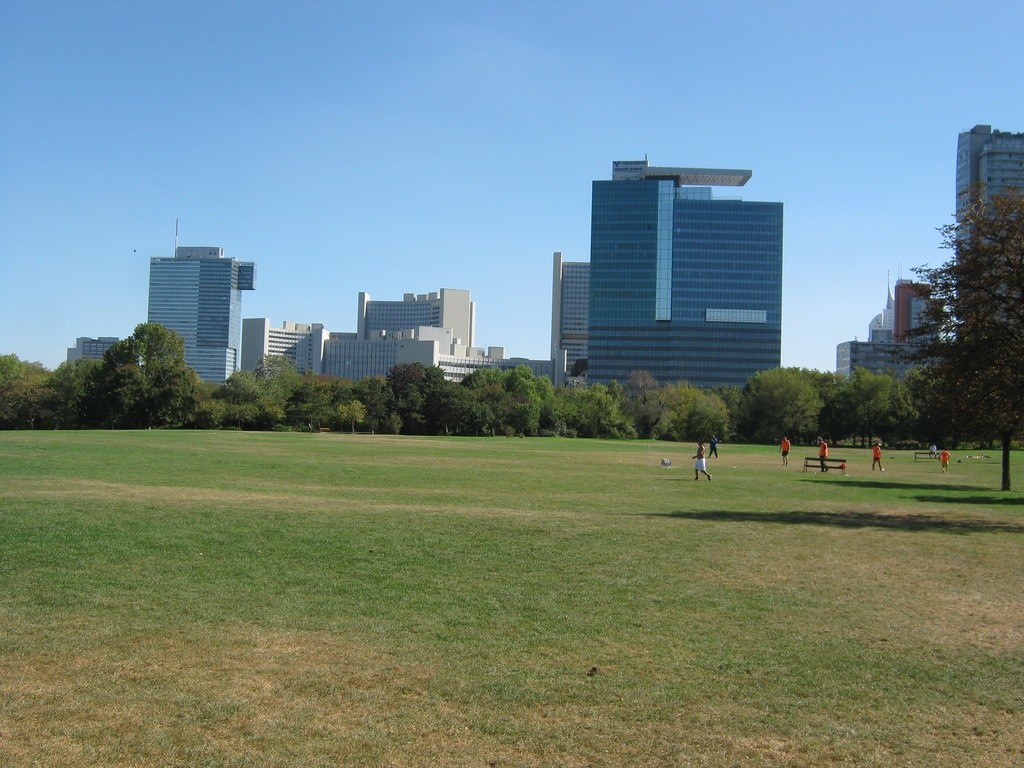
[825,468,829,472]
[880,469,884,471]
[708,475,712,481]
[695,478,699,480]
[716,456,719,460]
[820,469,824,472]
[942,469,945,473]
[707,455,711,458]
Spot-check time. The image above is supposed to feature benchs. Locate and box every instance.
[915,452,941,461]
[803,457,846,474]
[320,428,329,432]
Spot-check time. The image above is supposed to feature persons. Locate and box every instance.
[707,434,718,459]
[940,449,950,473]
[779,436,790,466]
[929,443,937,458]
[872,443,884,471]
[818,439,829,472]
[692,439,712,481]
